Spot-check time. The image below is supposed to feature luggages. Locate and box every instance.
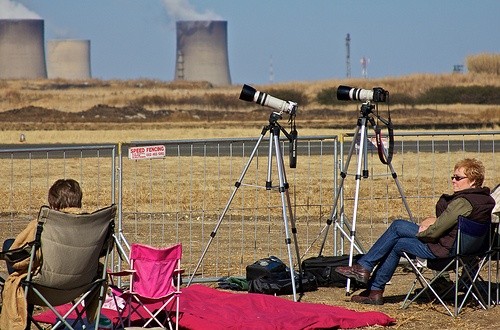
[302,253,365,287]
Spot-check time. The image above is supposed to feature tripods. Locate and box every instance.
[185,123,304,303]
[293,116,415,297]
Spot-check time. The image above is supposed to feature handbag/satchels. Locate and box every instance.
[247,256,288,280]
[249,274,318,294]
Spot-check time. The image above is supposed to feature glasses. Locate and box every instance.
[451,176,467,181]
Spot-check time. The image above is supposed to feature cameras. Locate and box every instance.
[337,85,386,103]
[238,83,298,116]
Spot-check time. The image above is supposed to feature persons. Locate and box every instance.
[335,158,496,305]
[2,179,88,281]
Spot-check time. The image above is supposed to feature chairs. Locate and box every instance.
[401,210,500,318]
[109,242,185,330]
[0,205,117,330]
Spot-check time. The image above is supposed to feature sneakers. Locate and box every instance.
[352,290,383,305]
[335,265,369,283]
